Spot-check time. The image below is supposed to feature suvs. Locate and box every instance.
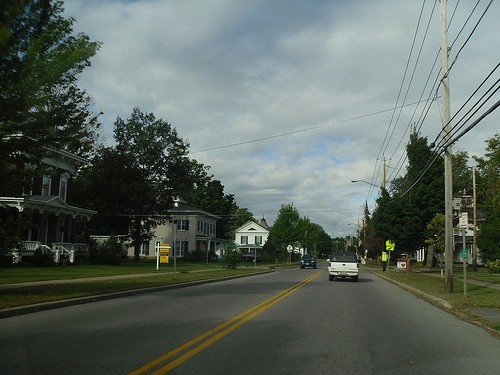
[327,254,359,282]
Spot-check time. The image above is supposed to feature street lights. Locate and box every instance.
[351,179,386,191]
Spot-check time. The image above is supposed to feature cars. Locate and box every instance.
[299,255,317,269]
